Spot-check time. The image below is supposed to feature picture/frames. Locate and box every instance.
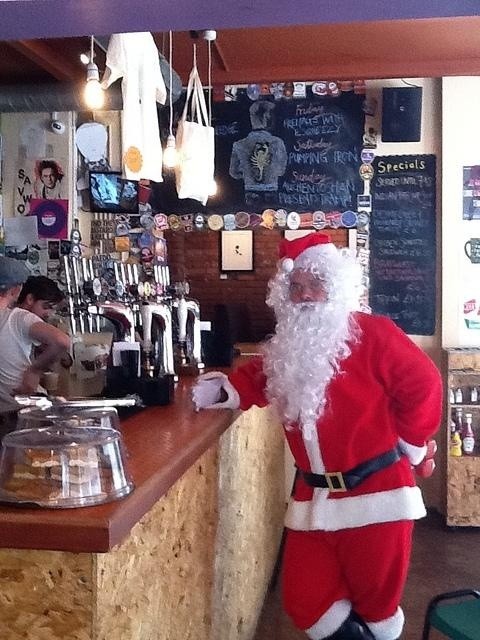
[217,228,256,276]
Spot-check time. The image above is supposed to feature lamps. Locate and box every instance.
[79,36,108,114]
[160,34,182,172]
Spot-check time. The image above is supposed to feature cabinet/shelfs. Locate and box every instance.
[442,343,480,533]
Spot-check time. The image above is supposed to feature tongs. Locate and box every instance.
[14,394,143,409]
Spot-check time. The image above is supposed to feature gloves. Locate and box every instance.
[191,375,228,413]
[398,436,427,466]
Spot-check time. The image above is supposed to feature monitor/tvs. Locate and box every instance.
[89,171,139,214]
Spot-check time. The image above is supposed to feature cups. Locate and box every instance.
[44,372,59,391]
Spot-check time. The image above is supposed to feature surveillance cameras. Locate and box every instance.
[51,120,65,135]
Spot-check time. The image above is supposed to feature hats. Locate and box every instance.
[276,233,336,273]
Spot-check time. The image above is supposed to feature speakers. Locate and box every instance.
[381,86,422,142]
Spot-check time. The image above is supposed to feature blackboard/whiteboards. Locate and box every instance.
[138,81,366,217]
[368,154,437,336]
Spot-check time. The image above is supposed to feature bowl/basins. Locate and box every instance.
[15,408,121,444]
[1,425,137,511]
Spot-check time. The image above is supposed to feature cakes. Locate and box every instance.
[1,446,117,505]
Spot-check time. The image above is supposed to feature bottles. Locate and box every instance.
[462,414,476,456]
[449,387,456,404]
[454,407,464,431]
[455,388,465,405]
[451,432,461,458]
[470,387,478,405]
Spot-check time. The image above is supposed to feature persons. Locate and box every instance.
[0,257,72,447]
[14,276,66,322]
[229,100,288,206]
[190,232,444,640]
[38,160,64,199]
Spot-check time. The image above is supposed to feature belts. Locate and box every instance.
[294,444,401,492]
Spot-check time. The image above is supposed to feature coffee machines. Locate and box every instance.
[133,299,180,387]
[167,296,209,373]
[101,302,131,388]
